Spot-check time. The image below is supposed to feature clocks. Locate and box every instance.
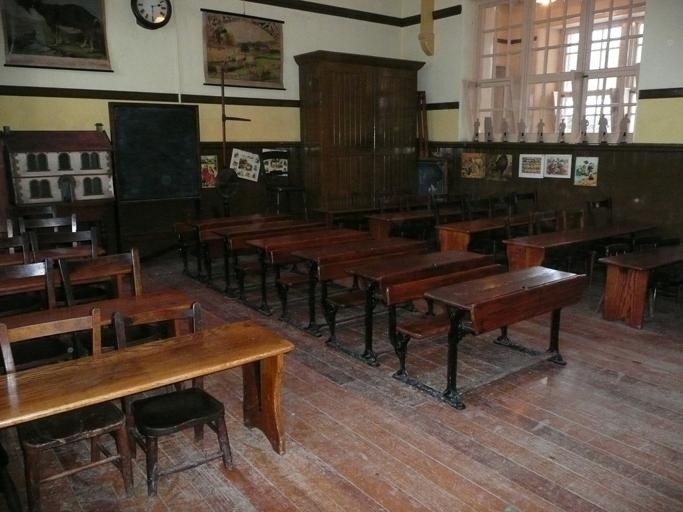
[130,0,172,29]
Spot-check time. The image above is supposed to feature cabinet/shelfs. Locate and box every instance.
[293,49,426,229]
[416,156,448,205]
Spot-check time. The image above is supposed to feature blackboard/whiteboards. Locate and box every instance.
[108,101,203,203]
